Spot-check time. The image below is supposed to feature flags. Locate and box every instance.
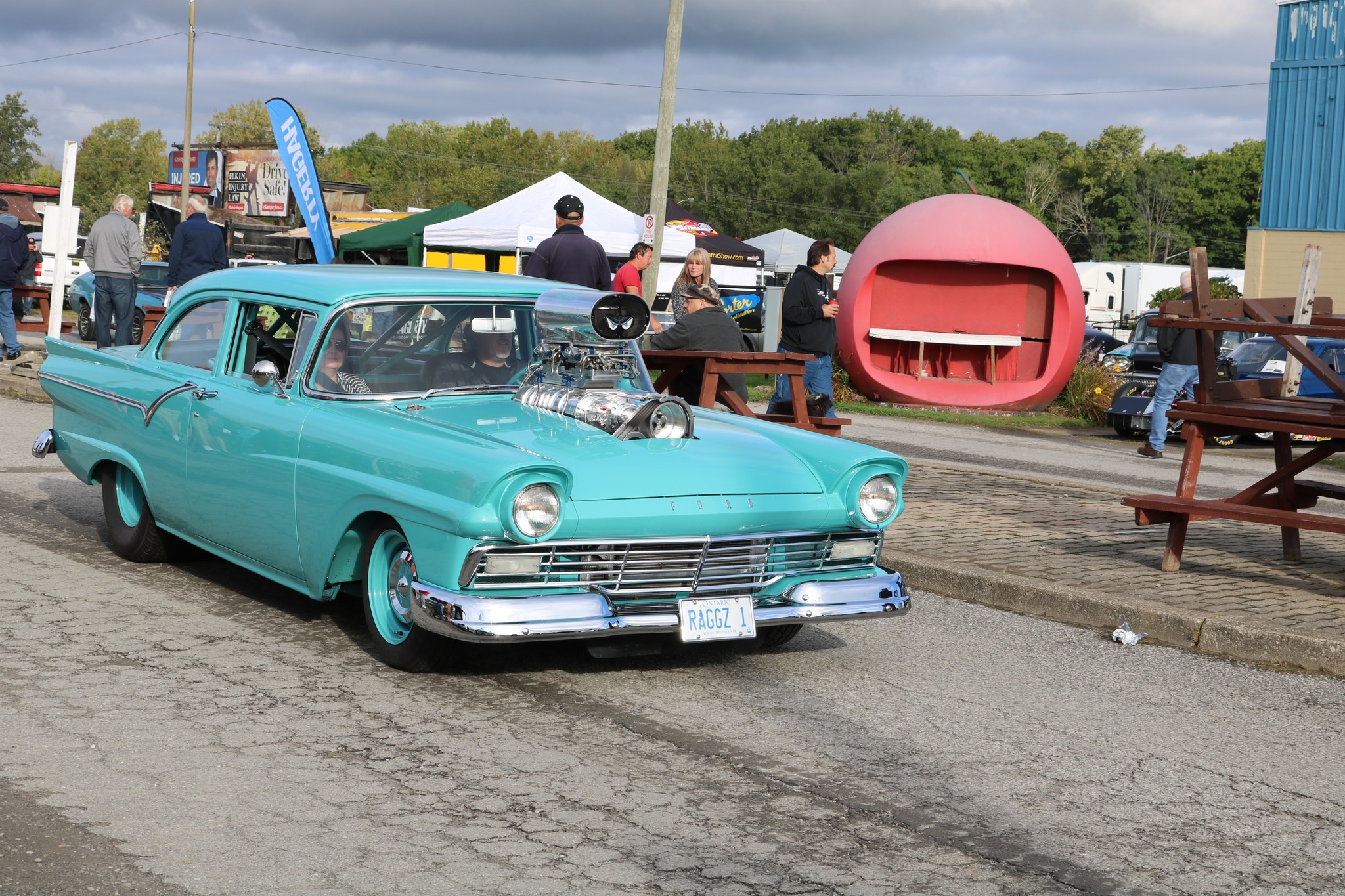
[265,97,337,264]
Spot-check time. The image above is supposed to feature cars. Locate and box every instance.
[27,232,289,344]
[1080,308,1345,449]
[31,266,913,672]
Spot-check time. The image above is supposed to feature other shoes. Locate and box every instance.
[1137,443,1162,458]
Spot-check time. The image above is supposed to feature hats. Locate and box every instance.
[553,194,584,220]
[679,283,720,305]
[0,198,8,211]
[28,237,36,244]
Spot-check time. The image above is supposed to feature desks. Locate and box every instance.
[869,327,1022,386]
[11,285,51,336]
[640,349,820,432]
[139,305,268,344]
[1147,245,1345,572]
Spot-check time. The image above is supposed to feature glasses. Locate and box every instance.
[323,338,347,352]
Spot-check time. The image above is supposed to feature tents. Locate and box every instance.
[337,202,478,265]
[422,171,852,297]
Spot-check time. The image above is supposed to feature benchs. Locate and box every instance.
[1288,315,1345,326]
[1293,480,1345,500]
[21,316,76,334]
[1122,493,1345,534]
[745,413,852,438]
[1148,319,1345,338]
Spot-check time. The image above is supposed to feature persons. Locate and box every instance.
[1137,272,1224,459]
[520,194,840,419]
[0,195,230,360]
[312,252,527,394]
[205,149,259,208]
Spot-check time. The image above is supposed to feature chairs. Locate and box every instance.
[418,351,471,391]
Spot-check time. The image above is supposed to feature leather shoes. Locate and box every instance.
[6,351,21,360]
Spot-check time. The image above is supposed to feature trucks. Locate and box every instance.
[1074,261,1244,333]
